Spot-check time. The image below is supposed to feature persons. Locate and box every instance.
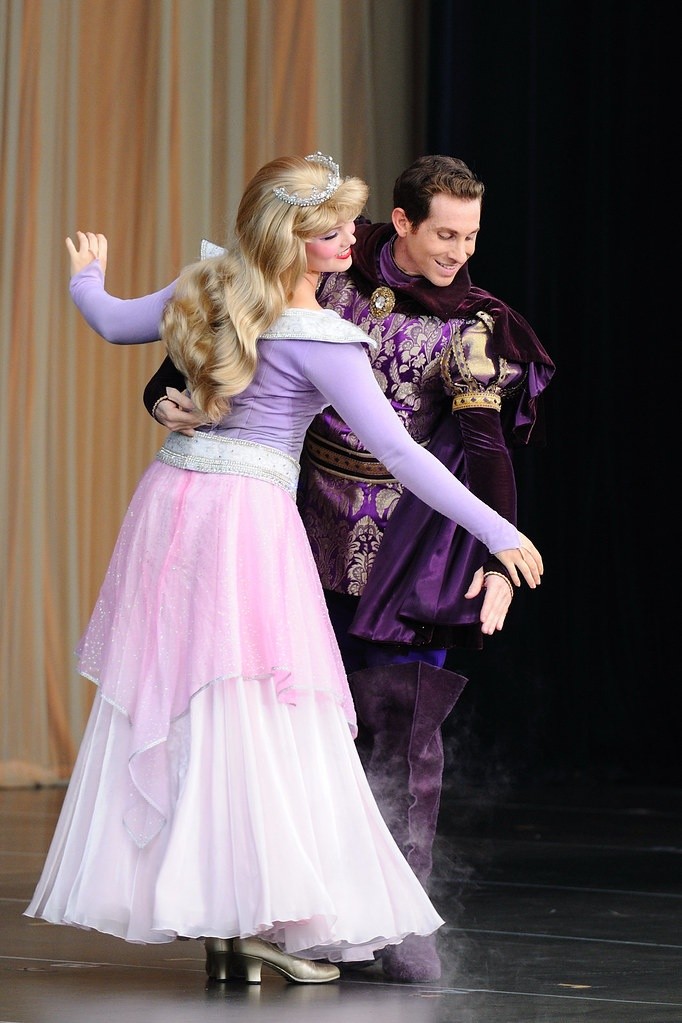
[143,155,556,982]
[23,152,544,983]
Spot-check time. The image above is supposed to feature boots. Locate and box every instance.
[338,661,467,982]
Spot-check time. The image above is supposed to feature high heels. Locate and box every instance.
[234,937,341,984]
[205,937,232,979]
